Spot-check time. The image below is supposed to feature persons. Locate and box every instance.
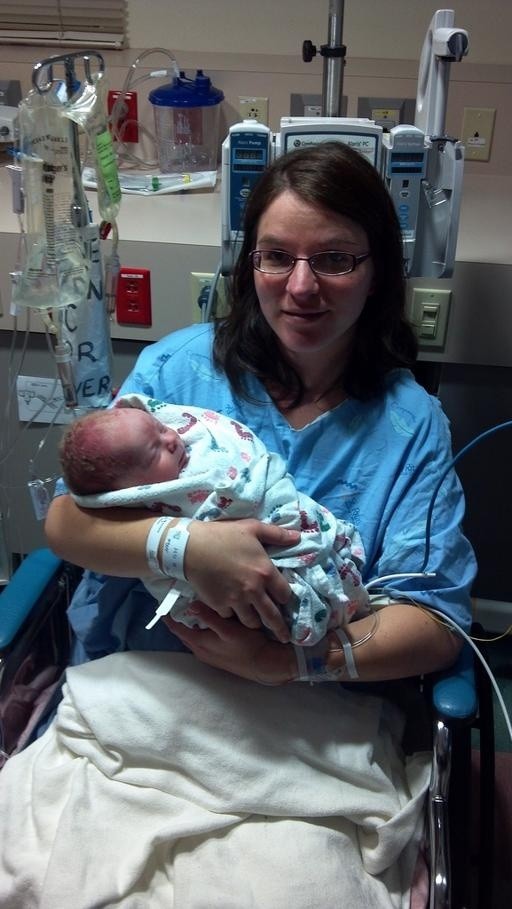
[2,142,478,907]
[56,404,193,497]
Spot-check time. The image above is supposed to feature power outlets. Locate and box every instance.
[116,267,150,326]
[191,271,231,324]
[238,96,270,127]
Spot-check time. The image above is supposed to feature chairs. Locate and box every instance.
[0,546,495,909]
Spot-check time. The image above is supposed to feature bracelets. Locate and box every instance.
[145,515,177,578]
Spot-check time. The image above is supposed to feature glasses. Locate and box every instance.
[247,248,372,278]
